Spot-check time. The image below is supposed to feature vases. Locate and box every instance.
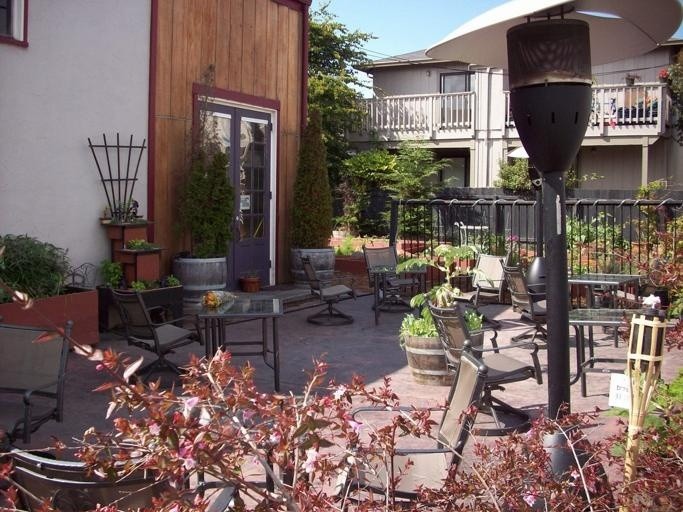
[626,77,634,85]
[659,77,667,82]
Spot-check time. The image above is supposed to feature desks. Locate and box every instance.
[568,308,644,397]
[568,272,642,348]
[198,296,286,396]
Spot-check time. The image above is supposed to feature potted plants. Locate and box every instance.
[240,269,262,295]
[98,198,168,287]
[0,234,101,349]
[290,107,336,290]
[169,148,236,307]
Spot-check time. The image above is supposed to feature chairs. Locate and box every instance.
[301,255,357,329]
[336,349,489,508]
[1,322,73,457]
[9,447,163,508]
[361,247,549,437]
[113,287,204,382]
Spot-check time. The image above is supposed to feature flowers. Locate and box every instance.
[656,68,670,79]
